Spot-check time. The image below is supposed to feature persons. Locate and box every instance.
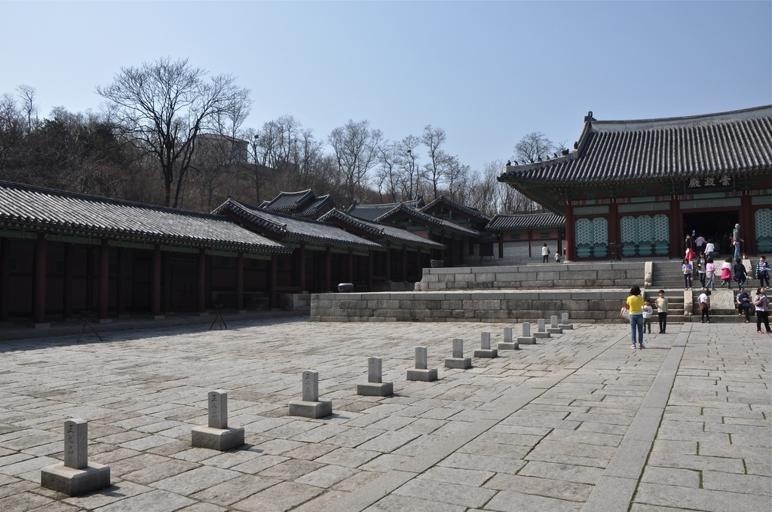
[541,242,550,264]
[554,251,561,263]
[654,289,668,334]
[624,284,645,349]
[641,298,653,334]
[682,224,772,335]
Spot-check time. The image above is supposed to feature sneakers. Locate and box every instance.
[756,329,772,335]
[644,329,665,334]
[631,343,646,349]
[700,319,710,323]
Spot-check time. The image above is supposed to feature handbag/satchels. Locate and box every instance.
[618,303,629,321]
